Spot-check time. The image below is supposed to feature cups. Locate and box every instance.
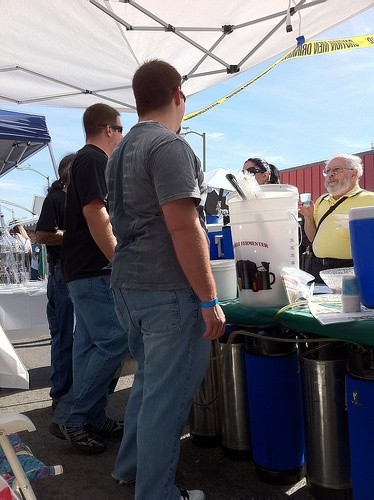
[341,294,360,313]
[299,193,312,208]
[209,259,238,302]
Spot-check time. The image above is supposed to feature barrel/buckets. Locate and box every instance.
[244,323,306,483]
[206,224,238,261]
[301,338,353,490]
[191,323,246,450]
[343,344,374,499]
[225,184,302,307]
[349,206,374,308]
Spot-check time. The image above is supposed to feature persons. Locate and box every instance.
[298,152,374,284]
[36,155,77,417]
[105,59,228,500]
[48,104,125,455]
[241,156,281,187]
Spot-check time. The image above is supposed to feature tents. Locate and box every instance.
[0,0,374,110]
[0,109,60,186]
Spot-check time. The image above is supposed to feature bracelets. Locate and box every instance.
[199,299,218,308]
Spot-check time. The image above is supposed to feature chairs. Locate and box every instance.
[0,412,63,500]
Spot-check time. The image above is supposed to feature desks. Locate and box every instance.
[217,297,374,348]
[0,281,52,389]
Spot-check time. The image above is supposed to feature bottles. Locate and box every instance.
[342,274,359,295]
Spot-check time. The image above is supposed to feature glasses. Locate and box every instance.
[101,124,122,133]
[242,167,263,175]
[322,166,354,176]
[173,87,186,102]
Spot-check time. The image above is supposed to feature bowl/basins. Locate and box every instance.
[319,267,354,294]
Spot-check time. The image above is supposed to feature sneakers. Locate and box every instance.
[49,422,106,453]
[179,489,206,500]
[111,470,135,484]
[85,418,125,437]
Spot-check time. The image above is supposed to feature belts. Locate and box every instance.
[313,257,353,266]
